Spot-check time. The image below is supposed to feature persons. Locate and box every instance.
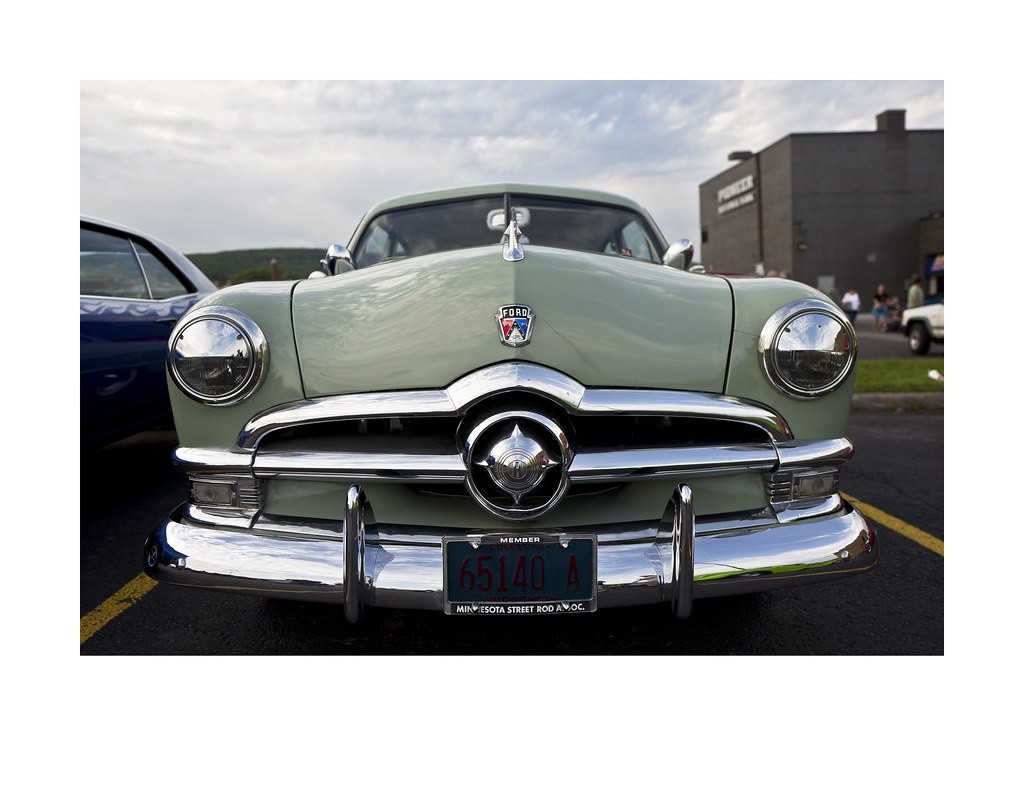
[889,295,905,331]
[927,255,944,302]
[842,284,863,329]
[872,284,889,332]
[905,273,926,310]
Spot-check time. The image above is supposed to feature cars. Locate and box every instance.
[79,214,219,532]
[139,183,876,623]
[900,302,944,356]
